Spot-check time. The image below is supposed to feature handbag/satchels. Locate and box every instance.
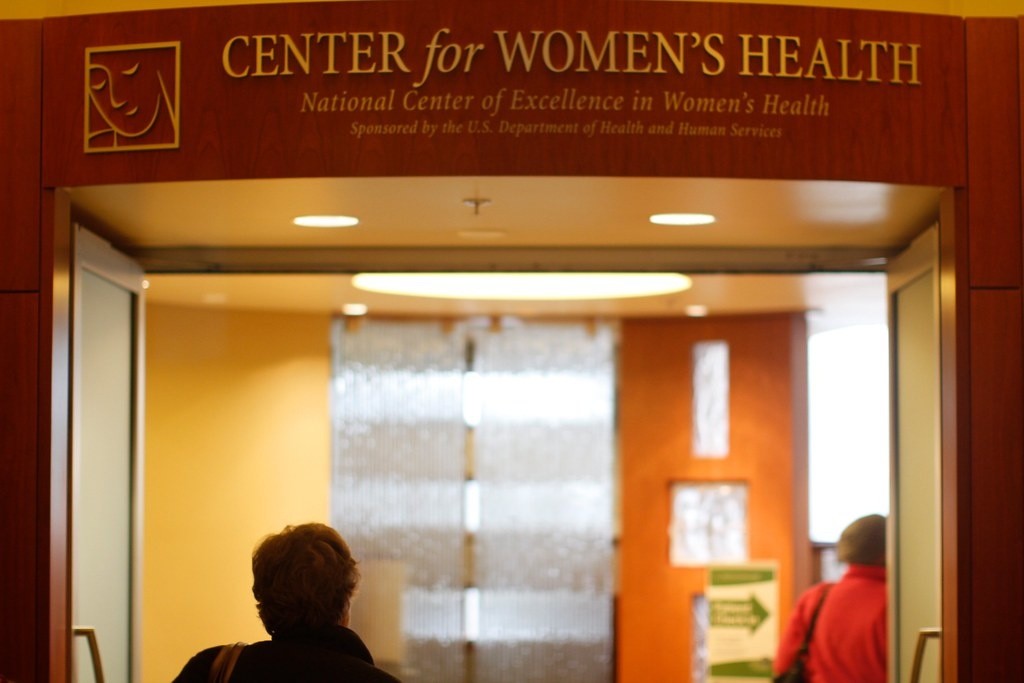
[772,582,833,683]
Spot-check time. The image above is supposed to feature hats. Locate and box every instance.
[835,515,886,563]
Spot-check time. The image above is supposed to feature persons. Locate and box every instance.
[172,523,404,683]
[773,514,888,683]
